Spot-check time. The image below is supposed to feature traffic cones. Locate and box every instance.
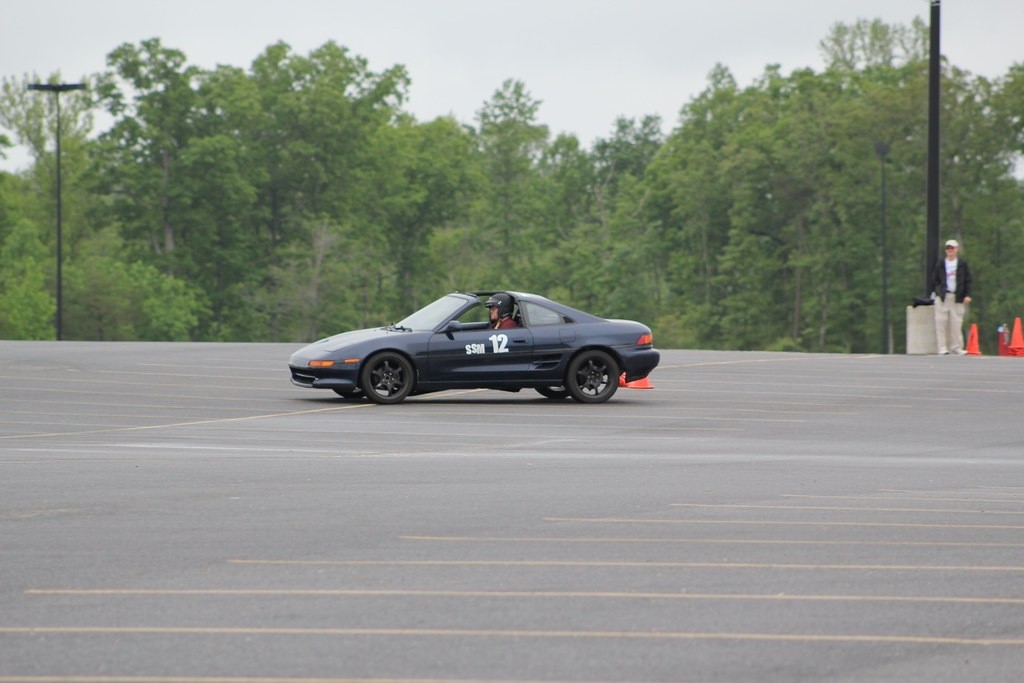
[1007,317,1024,357]
[965,324,981,355]
[628,375,655,390]
[618,371,626,387]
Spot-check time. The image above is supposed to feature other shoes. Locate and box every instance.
[938,350,949,355]
[952,348,967,355]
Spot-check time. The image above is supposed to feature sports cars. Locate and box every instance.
[290,289,660,406]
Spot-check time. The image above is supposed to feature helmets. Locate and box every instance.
[484,293,514,318]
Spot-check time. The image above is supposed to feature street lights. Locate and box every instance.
[28,81,92,342]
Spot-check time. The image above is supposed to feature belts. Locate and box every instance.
[946,291,956,294]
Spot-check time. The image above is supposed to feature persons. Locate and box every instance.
[485,293,517,330]
[930,239,975,355]
[998,322,1009,345]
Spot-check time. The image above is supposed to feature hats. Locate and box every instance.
[944,240,958,247]
